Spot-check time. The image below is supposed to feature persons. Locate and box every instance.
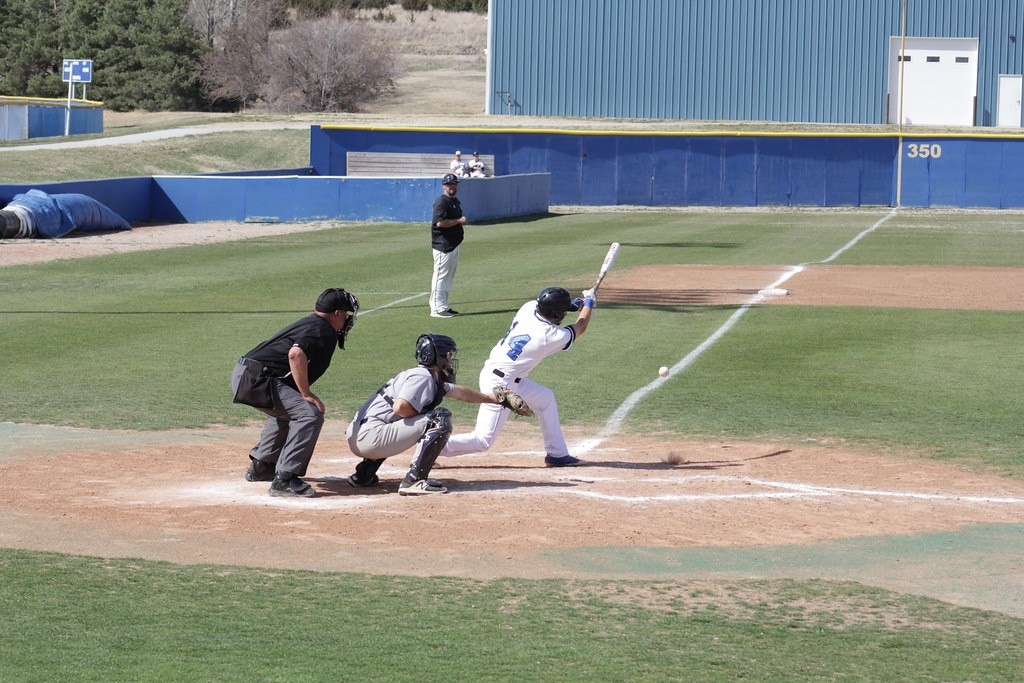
[345,333,519,495]
[442,286,620,465]
[450,150,469,178]
[430,174,468,318]
[469,151,484,178]
[230,287,359,498]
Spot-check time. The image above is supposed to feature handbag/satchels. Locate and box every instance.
[233,366,276,409]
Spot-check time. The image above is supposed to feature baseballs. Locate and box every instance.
[659,367,669,377]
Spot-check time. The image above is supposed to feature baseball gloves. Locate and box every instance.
[492,385,534,418]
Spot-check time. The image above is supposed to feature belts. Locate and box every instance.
[359,419,368,426]
[238,359,262,369]
[492,369,521,384]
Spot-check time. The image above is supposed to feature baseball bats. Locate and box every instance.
[578,242,621,311]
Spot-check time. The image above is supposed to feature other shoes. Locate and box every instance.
[447,309,459,316]
[545,454,586,468]
[410,460,441,469]
[430,310,454,318]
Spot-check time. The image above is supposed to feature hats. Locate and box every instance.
[314,287,355,313]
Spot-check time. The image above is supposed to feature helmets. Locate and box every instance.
[442,174,459,185]
[455,150,462,156]
[473,151,479,157]
[414,333,459,386]
[534,287,572,320]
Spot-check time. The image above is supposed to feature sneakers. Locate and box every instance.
[244,459,276,482]
[398,477,448,495]
[267,471,316,499]
[346,474,378,488]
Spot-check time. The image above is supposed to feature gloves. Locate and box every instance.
[570,297,585,311]
[582,287,596,307]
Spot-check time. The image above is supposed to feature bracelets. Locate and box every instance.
[584,299,593,307]
